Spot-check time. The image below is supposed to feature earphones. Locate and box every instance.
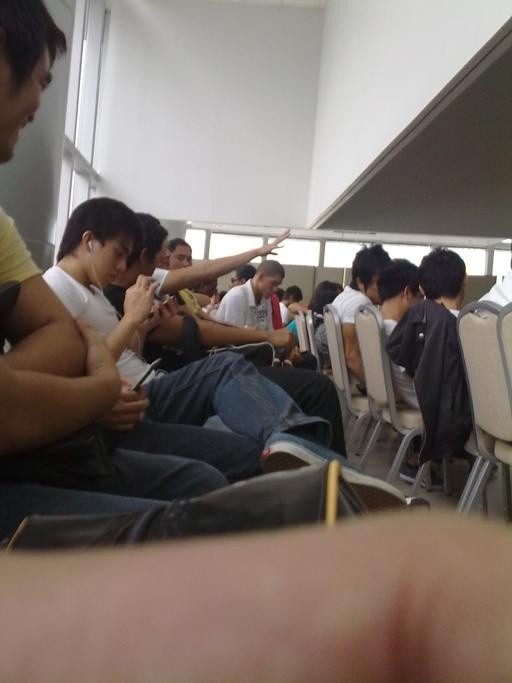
[87,241,92,254]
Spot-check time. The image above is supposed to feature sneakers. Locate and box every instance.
[259,432,408,514]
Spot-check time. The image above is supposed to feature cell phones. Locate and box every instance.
[151,296,176,313]
[131,357,162,391]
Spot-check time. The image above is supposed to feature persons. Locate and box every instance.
[0,0,512,683]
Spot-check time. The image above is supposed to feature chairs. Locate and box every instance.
[296,308,322,372]
[322,303,423,485]
[409,301,511,518]
